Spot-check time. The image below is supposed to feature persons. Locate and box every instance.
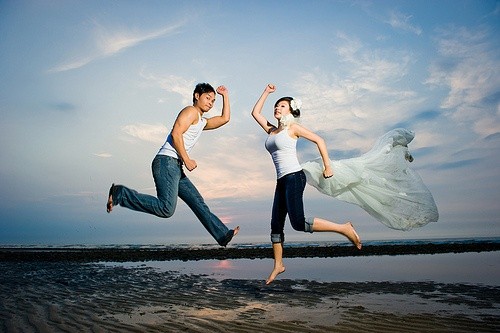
[107,83,241,248]
[252,83,363,284]
